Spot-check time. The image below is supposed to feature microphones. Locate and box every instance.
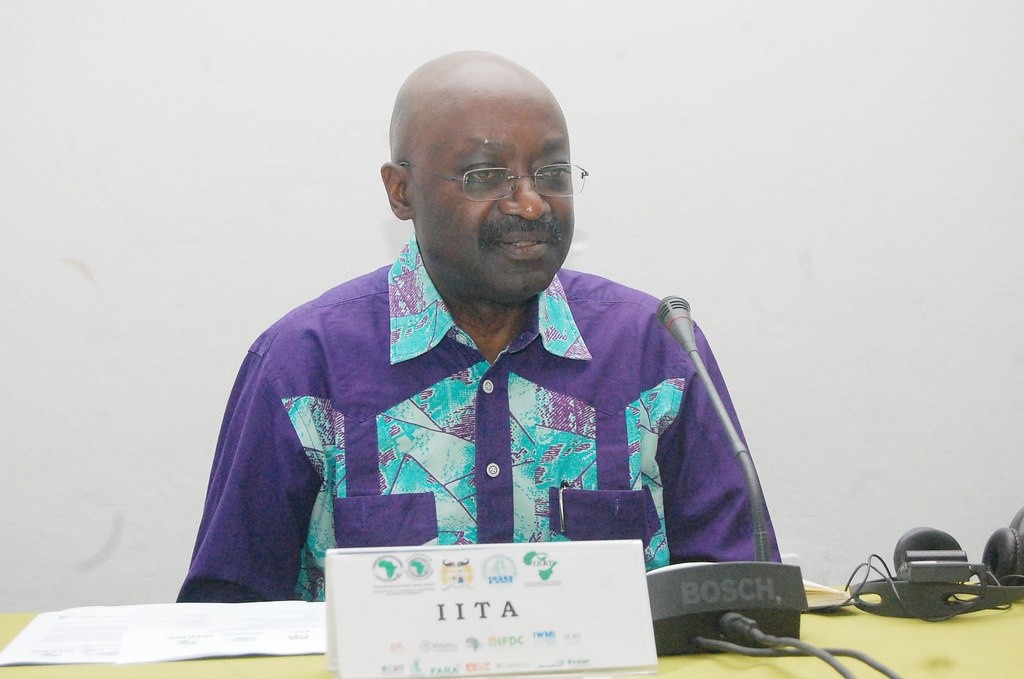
[644,294,809,655]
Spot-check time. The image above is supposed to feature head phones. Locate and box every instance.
[847,526,1024,621]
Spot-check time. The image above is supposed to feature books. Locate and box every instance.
[801,580,858,611]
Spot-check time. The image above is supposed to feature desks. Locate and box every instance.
[0,570,1024,679]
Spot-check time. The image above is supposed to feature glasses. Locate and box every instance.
[397,162,588,201]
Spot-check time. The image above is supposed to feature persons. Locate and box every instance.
[177,49,782,604]
